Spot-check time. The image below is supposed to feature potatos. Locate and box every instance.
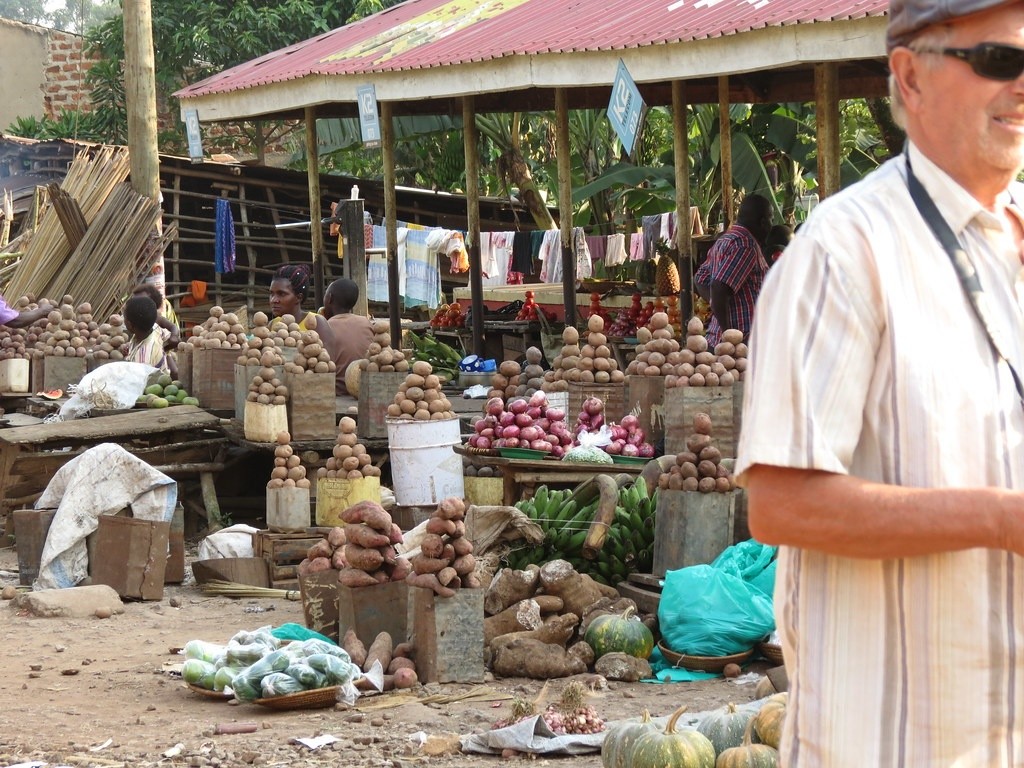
[317,414,381,477]
[389,363,455,420]
[360,320,409,371]
[724,663,741,676]
[661,412,737,492]
[0,292,130,359]
[300,497,652,687]
[489,310,749,400]
[268,431,311,487]
[179,307,335,404]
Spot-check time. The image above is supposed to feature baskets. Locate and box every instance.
[256,676,367,709]
[187,682,234,699]
[760,643,784,664]
[658,639,756,672]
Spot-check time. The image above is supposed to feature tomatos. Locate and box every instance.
[431,302,464,330]
[515,292,556,320]
[590,293,712,337]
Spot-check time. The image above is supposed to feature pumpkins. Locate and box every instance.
[345,360,361,399]
[602,690,791,767]
[585,606,653,659]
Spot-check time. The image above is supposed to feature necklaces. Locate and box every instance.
[905,138,1023,399]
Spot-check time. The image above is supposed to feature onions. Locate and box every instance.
[469,393,655,456]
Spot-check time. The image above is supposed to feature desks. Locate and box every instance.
[0,391,644,538]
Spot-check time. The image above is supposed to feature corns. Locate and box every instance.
[407,330,462,380]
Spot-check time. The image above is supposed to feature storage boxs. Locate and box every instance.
[0,346,752,687]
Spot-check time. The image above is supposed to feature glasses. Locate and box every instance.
[910,41,1024,80]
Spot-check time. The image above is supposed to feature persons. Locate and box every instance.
[122,283,180,379]
[318,278,375,397]
[693,194,775,344]
[735,0,1024,768]
[764,223,795,268]
[0,295,54,329]
[267,263,335,364]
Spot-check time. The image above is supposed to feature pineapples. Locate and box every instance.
[653,240,680,292]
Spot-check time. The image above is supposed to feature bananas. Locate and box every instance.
[509,478,661,583]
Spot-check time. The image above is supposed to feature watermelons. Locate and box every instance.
[37,389,63,399]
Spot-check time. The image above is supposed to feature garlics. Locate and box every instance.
[493,683,605,735]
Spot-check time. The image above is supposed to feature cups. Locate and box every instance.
[458,355,485,372]
[481,359,497,372]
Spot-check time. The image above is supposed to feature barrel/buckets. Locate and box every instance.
[385,414,465,506]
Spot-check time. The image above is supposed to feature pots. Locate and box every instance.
[459,372,497,388]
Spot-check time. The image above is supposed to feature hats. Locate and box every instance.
[885,0,1012,56]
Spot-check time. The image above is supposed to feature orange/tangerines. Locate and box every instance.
[138,375,199,408]
